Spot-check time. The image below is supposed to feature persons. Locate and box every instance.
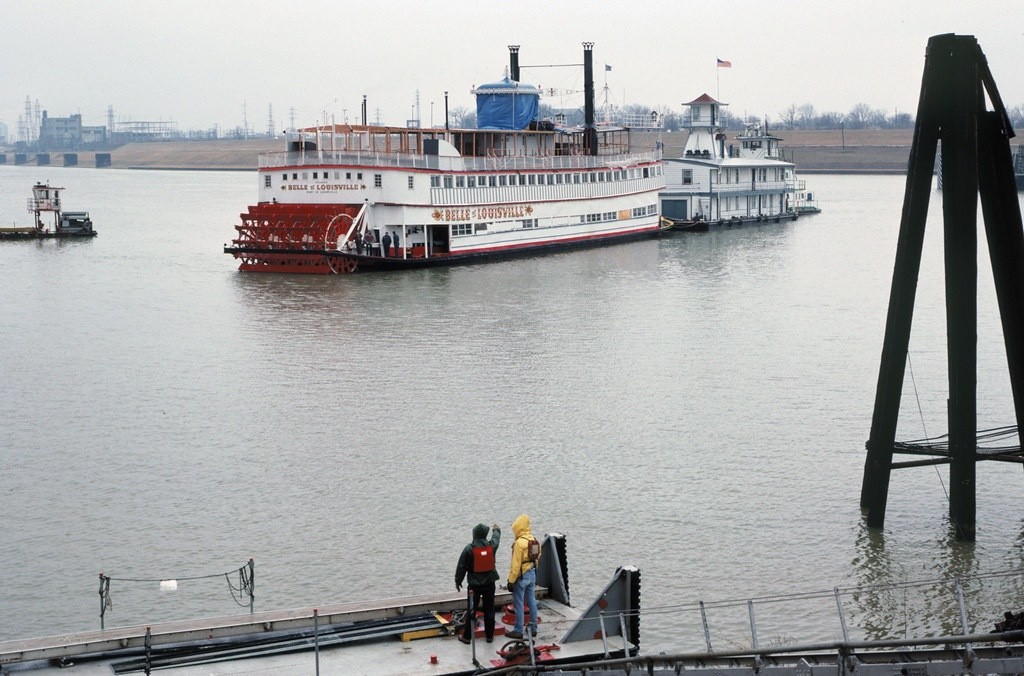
[504,513,541,639]
[354,230,362,256]
[382,232,391,257]
[455,522,502,645]
[363,230,373,256]
[392,230,399,257]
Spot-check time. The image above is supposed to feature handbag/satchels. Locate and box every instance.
[357,239,362,243]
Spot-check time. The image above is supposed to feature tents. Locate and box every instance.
[473,76,540,130]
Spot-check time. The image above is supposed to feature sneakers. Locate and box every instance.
[531,632,536,637]
[486,637,493,642]
[458,634,470,644]
[505,631,523,639]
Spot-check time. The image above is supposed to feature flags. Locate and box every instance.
[717,59,732,67]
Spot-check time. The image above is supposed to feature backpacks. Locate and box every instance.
[469,541,497,573]
[512,536,540,580]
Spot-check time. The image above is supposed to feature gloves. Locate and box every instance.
[507,582,514,592]
[456,581,463,592]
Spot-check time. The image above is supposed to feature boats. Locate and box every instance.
[2,529,1023,676]
[657,92,823,230]
[222,40,675,276]
[1,182,98,243]
